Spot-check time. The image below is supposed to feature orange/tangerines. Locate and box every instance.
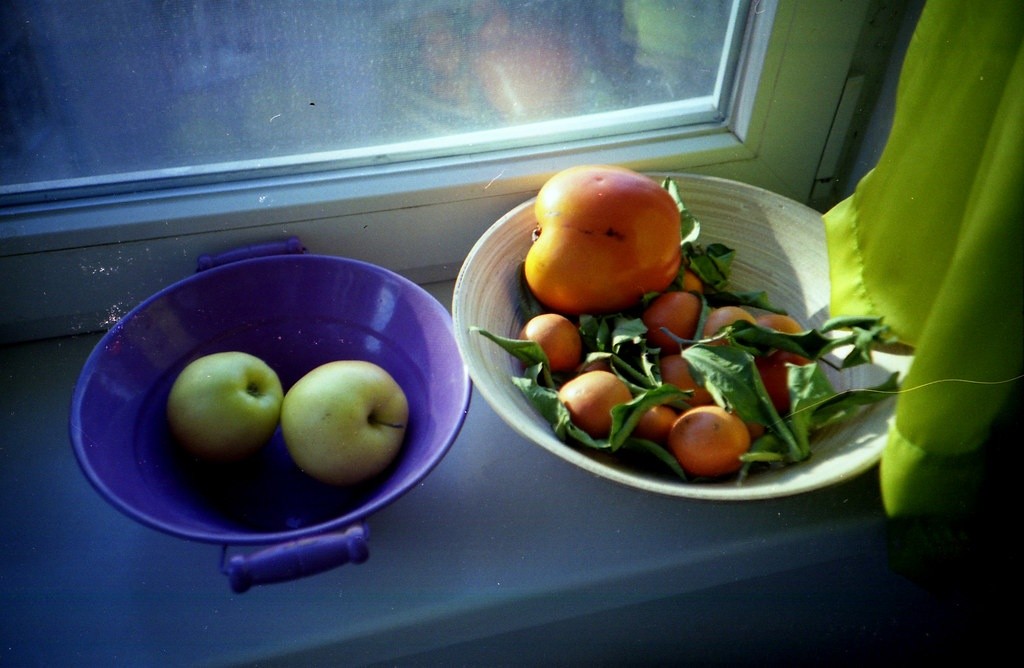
[518,273,810,474]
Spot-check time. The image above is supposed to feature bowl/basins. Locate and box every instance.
[71,240,469,589]
[453,173,912,499]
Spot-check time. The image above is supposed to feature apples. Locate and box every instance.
[280,359,408,484]
[168,351,284,459]
[523,163,683,311]
